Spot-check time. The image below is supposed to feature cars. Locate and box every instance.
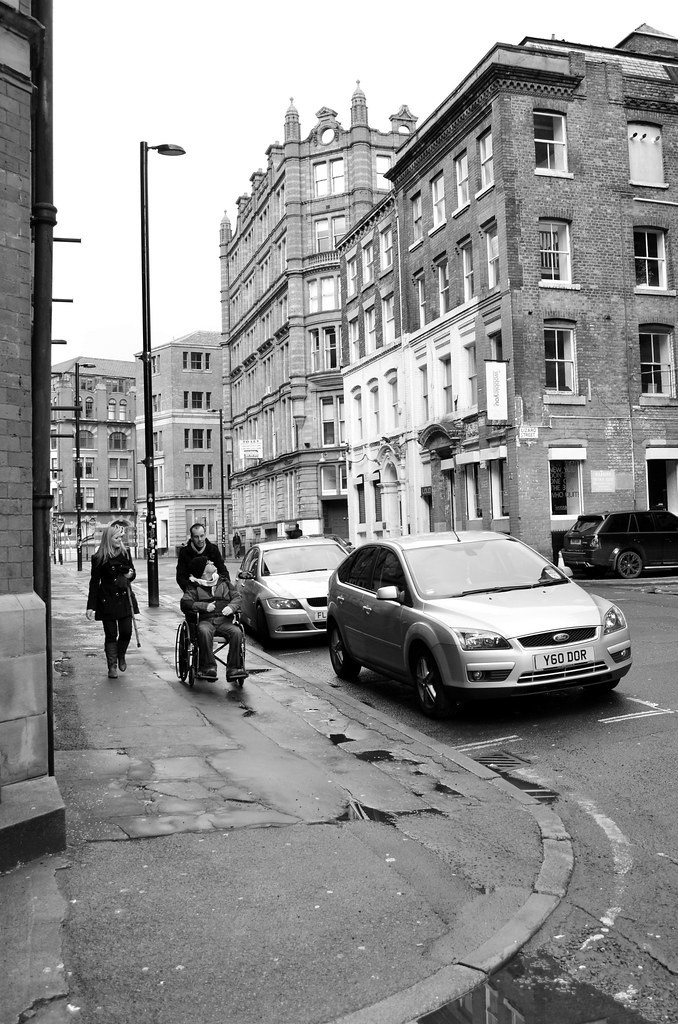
[325,531,632,722]
[231,537,354,650]
[302,535,354,555]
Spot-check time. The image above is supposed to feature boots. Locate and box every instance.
[105,641,118,678]
[117,640,127,672]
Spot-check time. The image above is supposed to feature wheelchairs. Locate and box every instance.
[174,611,248,687]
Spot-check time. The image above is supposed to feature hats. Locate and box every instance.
[189,556,208,579]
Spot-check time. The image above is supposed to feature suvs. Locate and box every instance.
[561,509,678,579]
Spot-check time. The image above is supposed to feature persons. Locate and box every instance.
[176,523,230,593]
[86,527,140,678]
[233,532,242,559]
[180,557,249,683]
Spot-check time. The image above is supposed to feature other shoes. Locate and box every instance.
[206,669,217,682]
[226,668,244,683]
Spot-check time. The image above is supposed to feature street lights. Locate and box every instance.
[138,140,188,607]
[208,408,227,561]
[74,361,95,572]
